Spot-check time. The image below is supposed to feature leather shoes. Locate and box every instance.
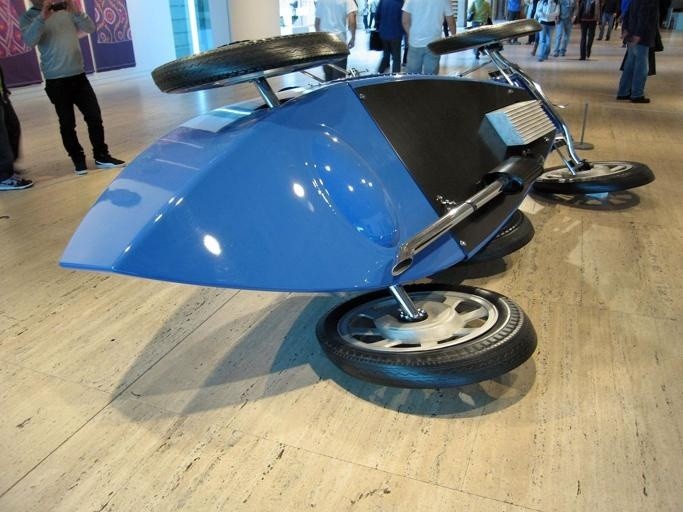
[616,96,649,102]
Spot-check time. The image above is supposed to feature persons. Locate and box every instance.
[0,71,34,190]
[311,0,456,76]
[468,1,664,103]
[18,0,126,176]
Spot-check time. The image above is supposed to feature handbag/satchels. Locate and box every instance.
[370,31,382,49]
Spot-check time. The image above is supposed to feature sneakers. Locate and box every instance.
[0,175,33,189]
[75,159,87,176]
[95,156,125,168]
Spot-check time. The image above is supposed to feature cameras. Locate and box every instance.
[51,1,66,11]
